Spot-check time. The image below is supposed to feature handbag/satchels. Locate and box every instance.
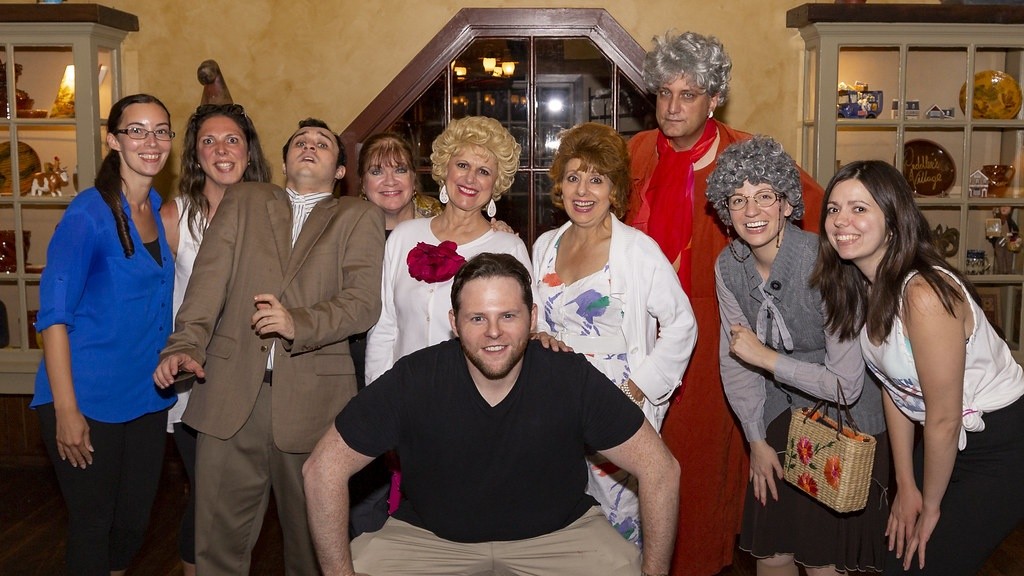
[783,375,876,513]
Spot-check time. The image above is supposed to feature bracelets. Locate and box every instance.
[619,380,645,408]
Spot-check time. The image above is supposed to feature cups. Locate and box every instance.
[28,309,43,348]
[980,163,1015,198]
[967,248,991,276]
[0,229,33,272]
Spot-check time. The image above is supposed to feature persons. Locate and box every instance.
[706,135,890,576]
[807,160,1024,576]
[30,93,177,576]
[160,102,273,576]
[365,116,573,518]
[627,32,825,576]
[155,118,386,576]
[349,134,513,537]
[532,122,698,549]
[302,254,681,576]
[991,207,1018,274]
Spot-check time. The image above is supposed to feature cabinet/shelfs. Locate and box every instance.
[1,2,138,394]
[786,3,1024,369]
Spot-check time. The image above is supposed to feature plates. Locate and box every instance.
[959,70,1022,119]
[16,109,48,118]
[0,141,42,197]
[893,138,957,197]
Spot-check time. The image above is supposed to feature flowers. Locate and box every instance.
[407,240,469,284]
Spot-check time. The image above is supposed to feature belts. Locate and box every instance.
[263,369,274,385]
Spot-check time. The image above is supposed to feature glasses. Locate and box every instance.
[106,127,175,145]
[193,104,250,151]
[725,188,781,210]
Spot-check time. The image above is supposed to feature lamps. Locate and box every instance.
[452,46,517,82]
